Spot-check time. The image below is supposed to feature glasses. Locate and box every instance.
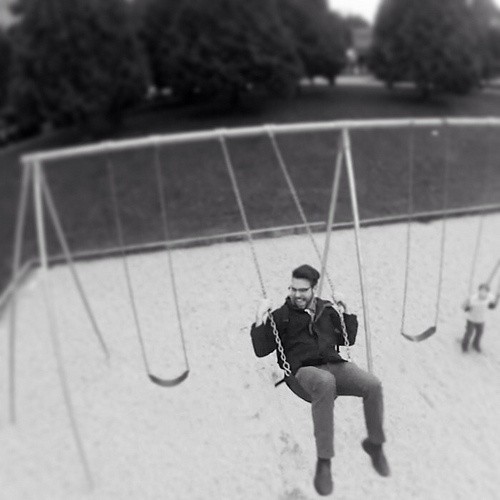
[288,285,312,292]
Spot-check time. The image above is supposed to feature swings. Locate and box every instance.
[399,122,450,343]
[462,125,500,311]
[101,148,191,387]
[218,132,357,404]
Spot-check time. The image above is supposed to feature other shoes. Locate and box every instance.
[314,460,333,496]
[362,438,390,476]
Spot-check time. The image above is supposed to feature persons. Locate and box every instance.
[460,283,499,353]
[251,264,391,495]
